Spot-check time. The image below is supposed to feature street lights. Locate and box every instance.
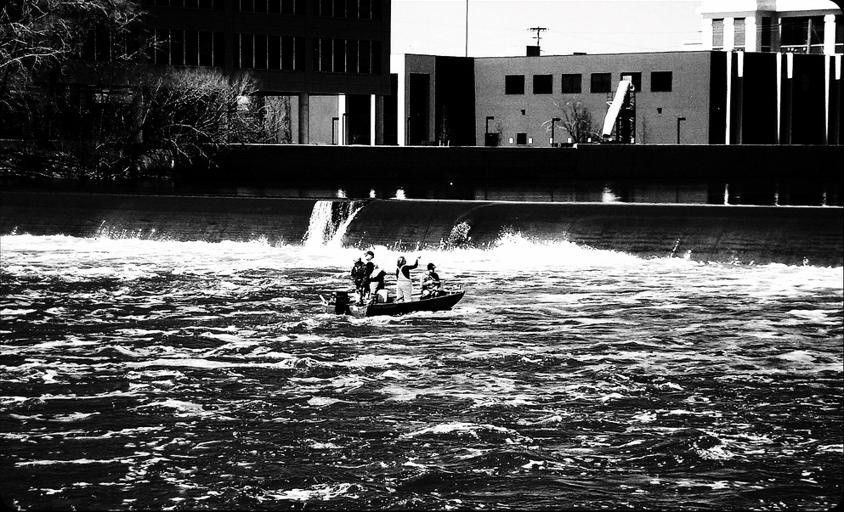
[676,115,685,202]
[485,116,494,200]
[332,116,339,145]
[551,117,561,202]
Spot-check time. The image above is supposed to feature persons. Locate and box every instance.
[395,256,421,302]
[351,251,375,306]
[369,265,387,295]
[420,263,446,299]
[601,184,622,203]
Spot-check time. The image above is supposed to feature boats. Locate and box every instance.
[308,281,467,319]
